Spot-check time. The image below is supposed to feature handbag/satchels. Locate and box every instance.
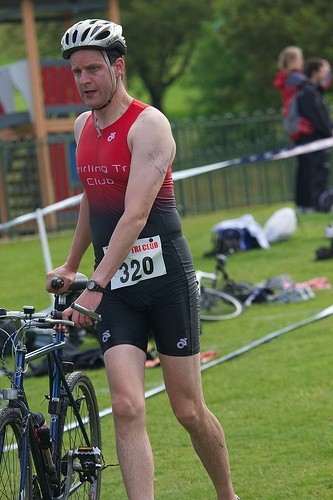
[284,83,315,140]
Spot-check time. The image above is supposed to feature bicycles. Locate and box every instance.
[195,253,274,321]
[0,271,119,500]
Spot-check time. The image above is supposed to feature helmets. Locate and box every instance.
[60,19,127,60]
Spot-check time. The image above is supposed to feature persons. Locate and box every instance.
[274,46,332,213]
[46,17,240,500]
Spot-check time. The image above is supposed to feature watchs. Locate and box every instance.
[86,280,105,293]
